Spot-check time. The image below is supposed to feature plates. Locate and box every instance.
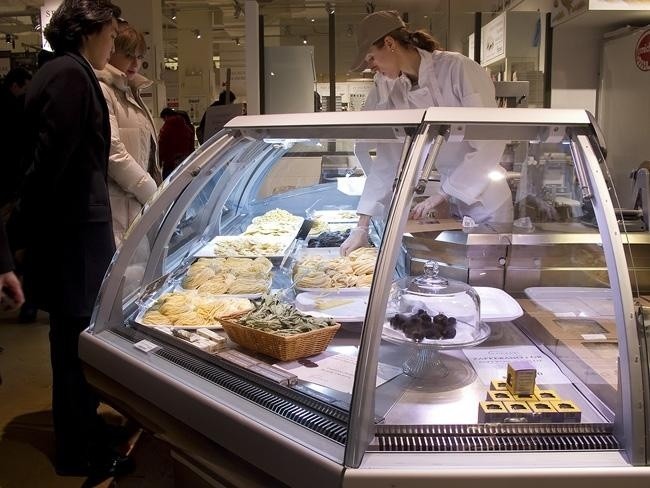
[240,215,305,241]
[524,286,617,320]
[381,313,491,394]
[295,286,523,323]
[290,246,395,293]
[191,235,296,265]
[135,292,257,329]
[173,270,273,299]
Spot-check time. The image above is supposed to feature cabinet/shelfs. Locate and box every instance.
[76,105,649,488]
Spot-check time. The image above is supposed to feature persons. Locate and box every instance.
[24,0,135,475]
[174,110,195,133]
[0,66,31,153]
[94,24,163,249]
[339,11,514,258]
[0,212,24,313]
[196,90,236,146]
[156,107,195,181]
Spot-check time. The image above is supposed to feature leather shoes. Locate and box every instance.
[54,416,136,475]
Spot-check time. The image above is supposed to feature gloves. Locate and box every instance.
[408,189,451,220]
[339,226,371,257]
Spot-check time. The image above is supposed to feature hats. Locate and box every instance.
[350,11,407,74]
